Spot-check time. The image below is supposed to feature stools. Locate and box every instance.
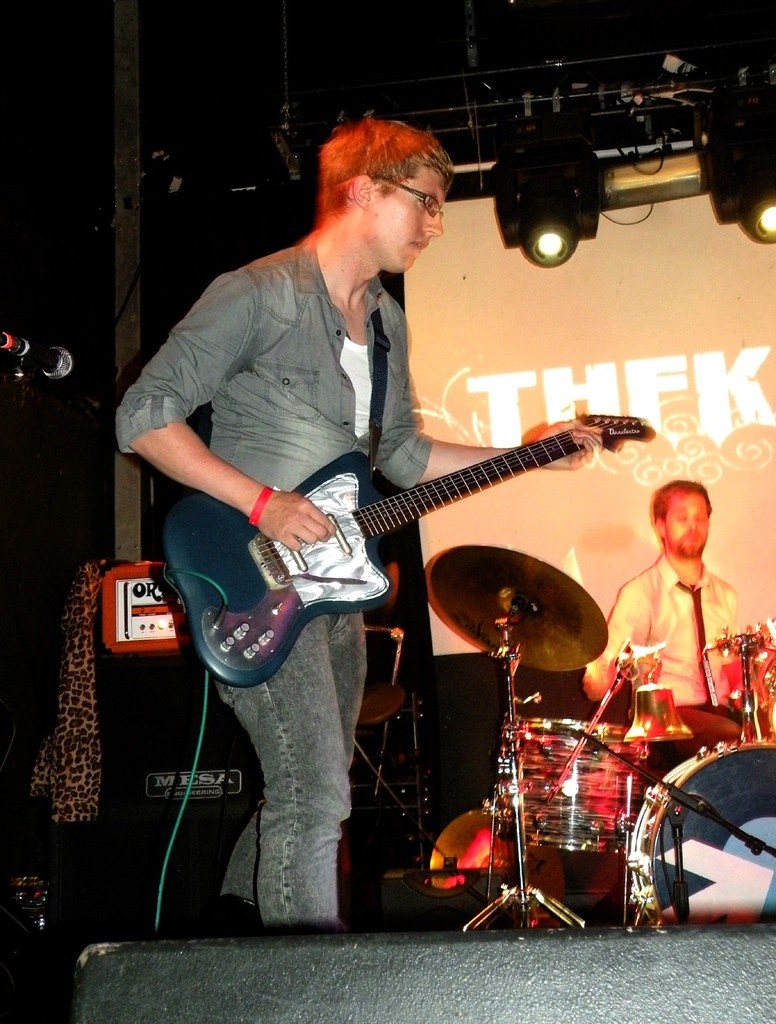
[352,682,408,810]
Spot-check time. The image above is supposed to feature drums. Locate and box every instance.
[627,742,776,930]
[492,717,646,851]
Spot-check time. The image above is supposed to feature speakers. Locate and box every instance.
[54,811,271,987]
[430,653,584,840]
[89,649,268,805]
[74,919,776,1024]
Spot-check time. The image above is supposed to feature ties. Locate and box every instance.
[676,581,719,707]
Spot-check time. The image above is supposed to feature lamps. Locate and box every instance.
[492,140,601,267]
[707,135,776,245]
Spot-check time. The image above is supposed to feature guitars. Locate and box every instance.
[148,413,647,685]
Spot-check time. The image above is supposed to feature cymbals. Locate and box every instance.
[430,811,563,932]
[676,706,743,756]
[426,545,607,672]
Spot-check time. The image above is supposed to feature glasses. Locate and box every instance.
[372,176,444,217]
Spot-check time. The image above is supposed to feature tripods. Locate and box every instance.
[462,620,585,932]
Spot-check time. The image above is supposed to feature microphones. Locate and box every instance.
[0,332,73,379]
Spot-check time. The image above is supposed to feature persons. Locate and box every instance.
[580,478,771,726]
[112,116,606,930]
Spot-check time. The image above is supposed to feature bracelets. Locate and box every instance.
[247,486,276,528]
